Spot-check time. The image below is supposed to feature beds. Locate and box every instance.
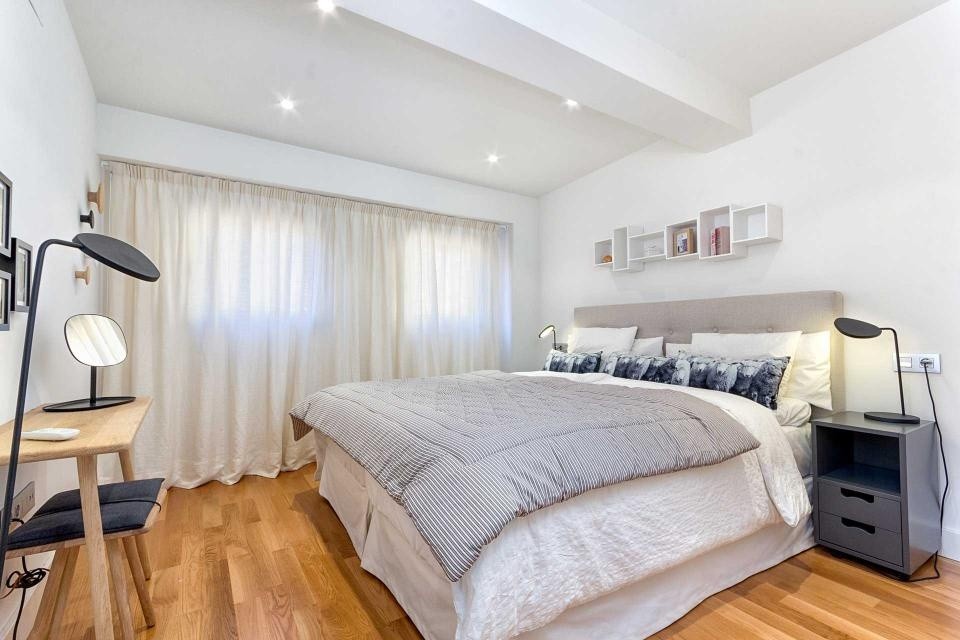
[289,289,847,640]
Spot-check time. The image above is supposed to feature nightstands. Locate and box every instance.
[810,411,942,581]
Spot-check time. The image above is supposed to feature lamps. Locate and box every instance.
[834,318,920,425]
[0,232,160,583]
[539,325,568,353]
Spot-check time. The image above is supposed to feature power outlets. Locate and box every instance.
[0,481,36,535]
[916,354,940,374]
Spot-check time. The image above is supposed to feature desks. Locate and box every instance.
[0,397,153,640]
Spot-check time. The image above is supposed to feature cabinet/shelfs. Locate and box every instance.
[593,202,783,273]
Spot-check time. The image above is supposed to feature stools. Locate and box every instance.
[0,478,167,640]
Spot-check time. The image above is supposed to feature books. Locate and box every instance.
[673,229,694,256]
[711,226,730,256]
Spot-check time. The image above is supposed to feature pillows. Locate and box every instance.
[542,326,834,411]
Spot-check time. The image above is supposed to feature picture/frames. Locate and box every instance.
[0,270,12,332]
[0,171,13,259]
[10,237,33,312]
[673,228,694,256]
